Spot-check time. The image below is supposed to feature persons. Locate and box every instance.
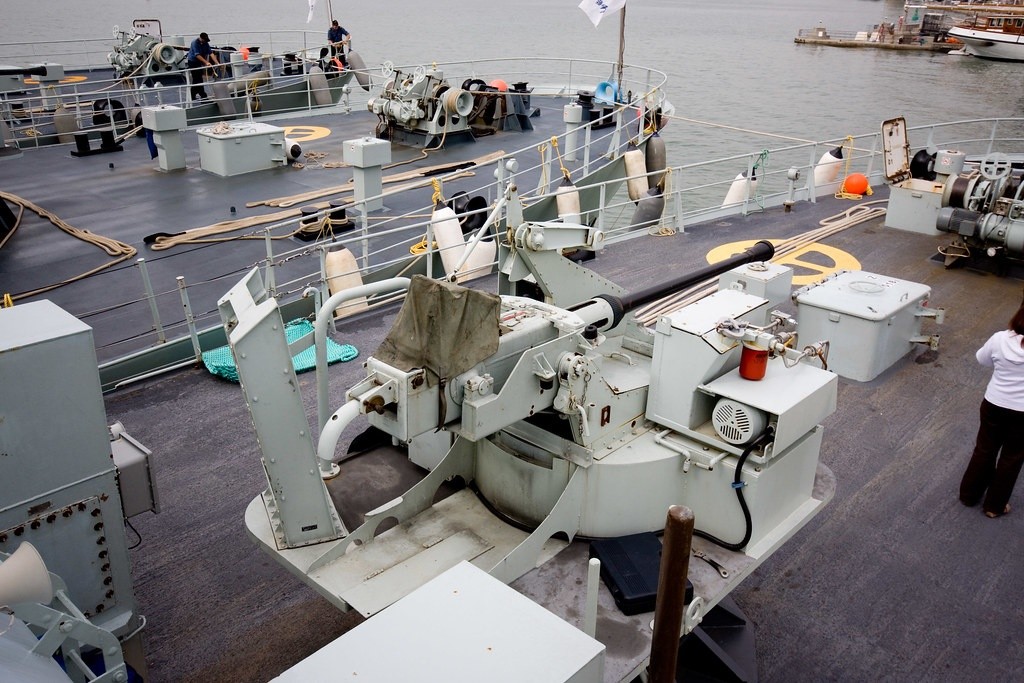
[188,33,221,101]
[959,300,1024,518]
[327,20,351,70]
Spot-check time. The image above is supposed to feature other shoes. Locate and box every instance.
[987,503,1010,518]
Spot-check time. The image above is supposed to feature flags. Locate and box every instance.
[307,0,318,22]
[579,0,626,28]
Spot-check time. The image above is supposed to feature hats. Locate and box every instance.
[200,32,210,42]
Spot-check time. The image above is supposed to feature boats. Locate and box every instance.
[947,23,1024,63]
[0,18,354,150]
[0,57,675,324]
